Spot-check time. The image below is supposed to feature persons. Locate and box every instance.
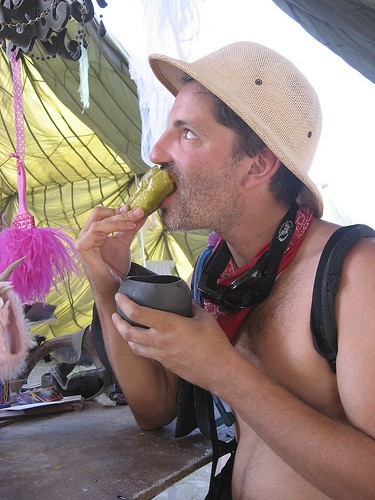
[72,40,374,500]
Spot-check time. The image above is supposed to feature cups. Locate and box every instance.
[116,275,193,329]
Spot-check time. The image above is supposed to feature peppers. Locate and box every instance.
[125,165,174,219]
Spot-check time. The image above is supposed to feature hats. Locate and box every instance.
[148,40,324,218]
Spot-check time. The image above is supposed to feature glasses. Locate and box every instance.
[40,360,111,402]
[196,202,301,313]
